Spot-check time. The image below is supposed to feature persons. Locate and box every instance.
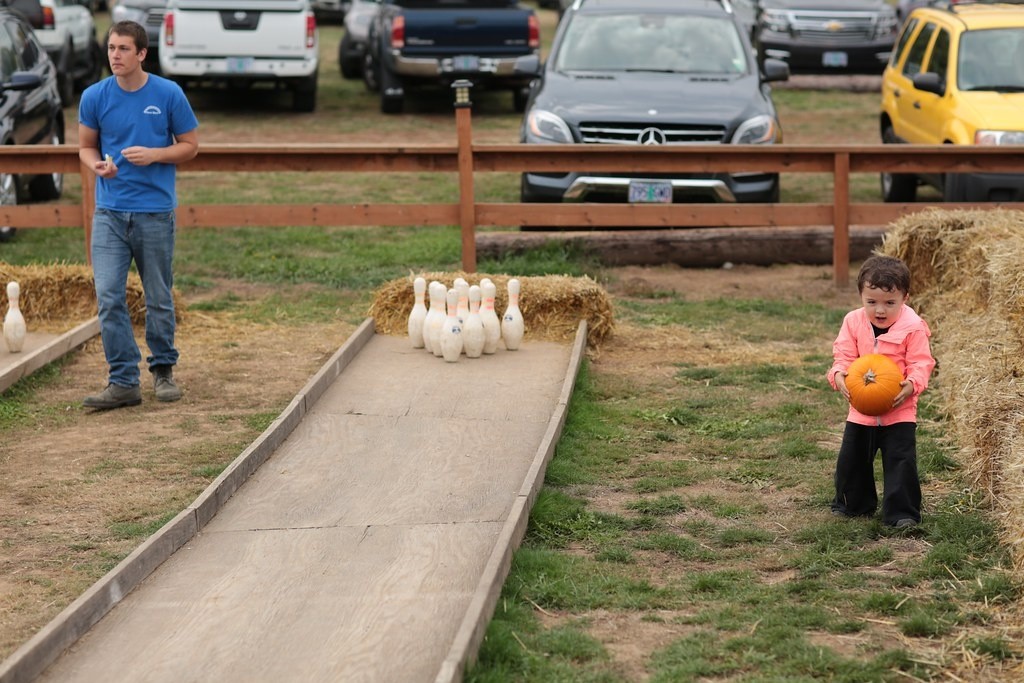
[76,21,201,407]
[827,257,935,527]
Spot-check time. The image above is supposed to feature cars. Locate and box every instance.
[336,1,382,80]
[111,1,166,48]
[749,0,901,81]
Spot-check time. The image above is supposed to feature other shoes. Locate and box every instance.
[830,506,853,520]
[895,516,915,531]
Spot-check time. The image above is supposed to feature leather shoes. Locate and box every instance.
[153,369,181,402]
[83,383,143,409]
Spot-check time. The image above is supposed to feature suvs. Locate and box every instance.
[0,1,102,109]
[520,0,784,232]
[877,3,1024,203]
[0,7,64,245]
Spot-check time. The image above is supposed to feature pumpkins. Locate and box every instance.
[844,355,905,416]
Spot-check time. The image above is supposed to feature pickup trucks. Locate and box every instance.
[359,0,542,115]
[159,0,319,110]
